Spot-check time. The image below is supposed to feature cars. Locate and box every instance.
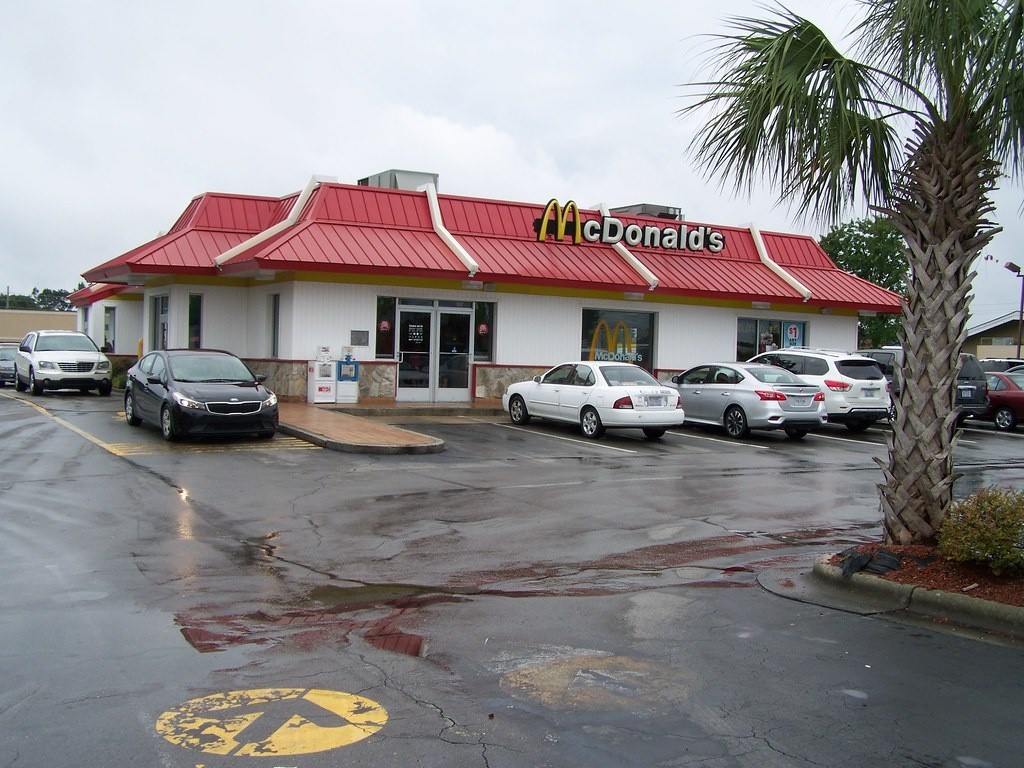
[125,348,279,442]
[966,357,1024,432]
[375,353,429,388]
[662,361,829,440]
[0,342,20,389]
[582,347,611,361]
[503,361,685,440]
[439,353,489,388]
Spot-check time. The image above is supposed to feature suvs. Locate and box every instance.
[14,329,113,396]
[713,346,892,433]
[853,346,990,427]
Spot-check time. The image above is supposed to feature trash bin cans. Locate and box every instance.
[307,352,336,404]
[336,353,359,404]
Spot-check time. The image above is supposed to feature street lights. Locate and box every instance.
[1003,261,1024,359]
